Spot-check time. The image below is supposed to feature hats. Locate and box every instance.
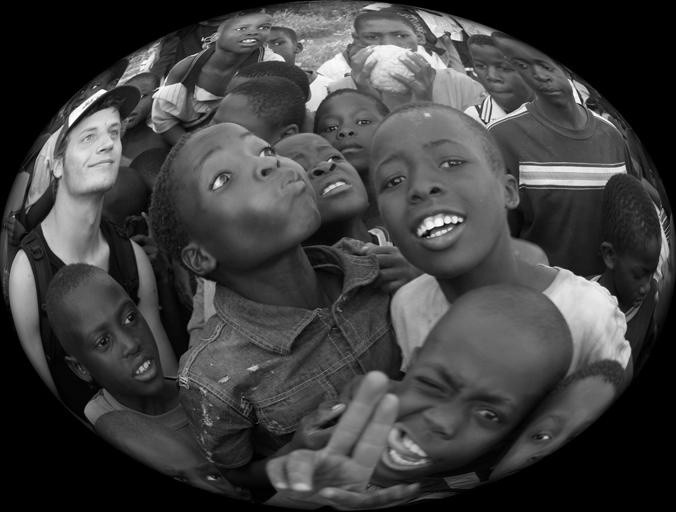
[47,84,142,160]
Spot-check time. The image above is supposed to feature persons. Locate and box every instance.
[0,0,675,511]
